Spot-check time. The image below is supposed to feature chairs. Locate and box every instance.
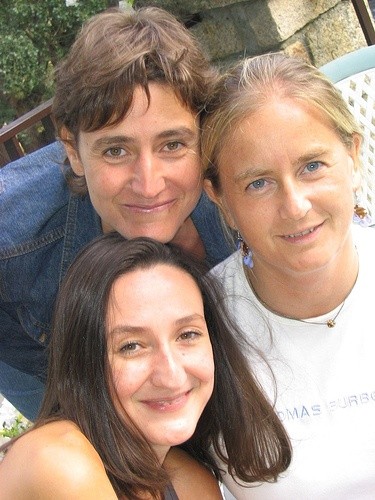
[318,45,375,228]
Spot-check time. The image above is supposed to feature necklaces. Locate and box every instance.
[246,244,359,328]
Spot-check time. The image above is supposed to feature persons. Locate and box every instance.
[199,52,375,499]
[0,233,293,500]
[0,7,242,423]
